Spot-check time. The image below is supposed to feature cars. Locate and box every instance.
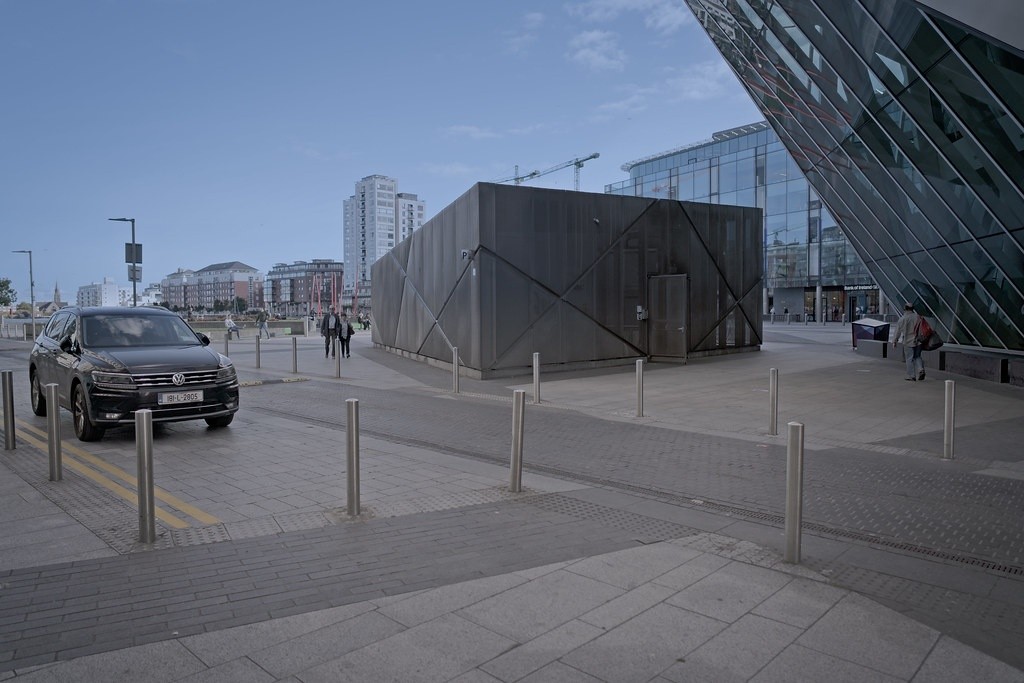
[274,312,286,320]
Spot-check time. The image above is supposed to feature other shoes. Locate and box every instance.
[918,370,926,380]
[332,356,335,359]
[325,355,328,358]
[905,375,916,381]
[347,354,350,358]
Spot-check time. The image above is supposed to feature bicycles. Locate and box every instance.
[309,314,319,326]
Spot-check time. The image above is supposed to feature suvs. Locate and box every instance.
[28,303,239,443]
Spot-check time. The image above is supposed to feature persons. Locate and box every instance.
[225,314,244,340]
[321,306,353,358]
[358,310,371,330]
[855,304,879,319]
[188,308,194,321]
[833,305,843,320]
[273,313,282,320]
[6,309,12,319]
[310,308,317,320]
[784,306,788,319]
[254,308,270,340]
[893,303,926,380]
[770,307,776,314]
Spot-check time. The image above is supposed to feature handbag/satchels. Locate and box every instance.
[914,315,943,350]
[347,325,355,335]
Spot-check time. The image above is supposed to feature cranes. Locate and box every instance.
[520,151,599,191]
[786,218,823,241]
[488,165,540,187]
[767,227,790,240]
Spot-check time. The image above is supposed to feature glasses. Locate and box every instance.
[330,308,334,310]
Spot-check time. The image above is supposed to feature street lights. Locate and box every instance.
[12,250,35,318]
[108,218,136,306]
